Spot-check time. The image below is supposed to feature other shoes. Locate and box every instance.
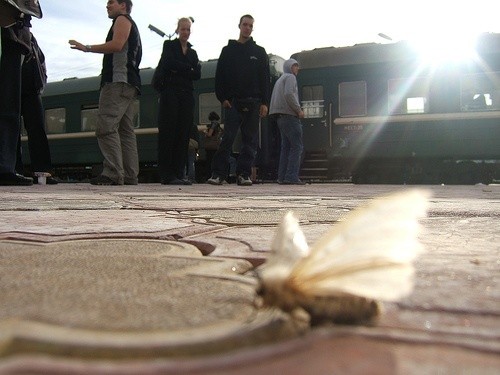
[33,176,58,184]
[283,178,306,184]
[10,173,33,185]
[208,176,223,184]
[237,173,252,185]
[167,178,192,185]
[90,175,115,185]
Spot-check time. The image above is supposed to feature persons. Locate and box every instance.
[157,17,201,186]
[269,58,307,185]
[0,0,58,185]
[207,14,270,186]
[69,0,143,186]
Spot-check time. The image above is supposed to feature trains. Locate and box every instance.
[20,33,500,183]
